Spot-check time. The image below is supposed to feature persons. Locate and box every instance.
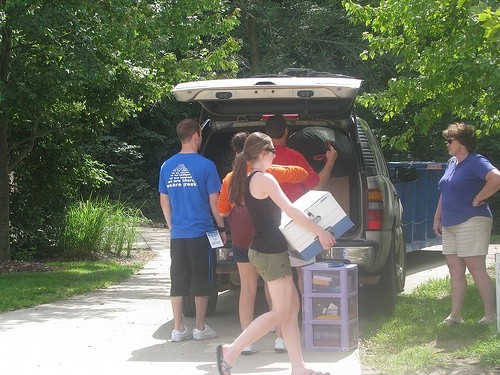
[158,118,229,342]
[216,132,337,374]
[431,122,500,328]
[216,131,308,355]
[264,114,338,332]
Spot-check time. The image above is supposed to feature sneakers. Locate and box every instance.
[193,324,217,340]
[274,337,287,352]
[241,342,258,355]
[171,326,193,342]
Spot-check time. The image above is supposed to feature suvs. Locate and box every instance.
[172,77,418,316]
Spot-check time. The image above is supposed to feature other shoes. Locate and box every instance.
[442,315,465,325]
[478,317,494,323]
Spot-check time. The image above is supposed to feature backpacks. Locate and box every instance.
[228,204,255,248]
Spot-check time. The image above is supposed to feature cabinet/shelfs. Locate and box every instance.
[302,263,358,352]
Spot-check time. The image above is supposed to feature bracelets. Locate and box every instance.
[474,196,481,203]
[218,227,225,233]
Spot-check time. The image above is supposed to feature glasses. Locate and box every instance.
[263,148,277,154]
[445,139,456,144]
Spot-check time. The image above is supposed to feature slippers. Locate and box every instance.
[216,344,232,375]
[307,369,330,375]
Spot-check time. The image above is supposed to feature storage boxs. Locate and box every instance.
[278,176,356,261]
